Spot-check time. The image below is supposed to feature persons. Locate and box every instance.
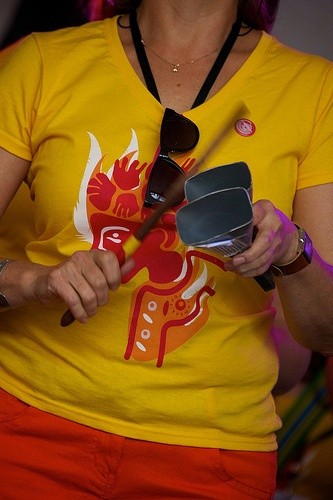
[0,0,332,499]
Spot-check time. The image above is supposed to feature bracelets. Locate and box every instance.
[1,258,19,312]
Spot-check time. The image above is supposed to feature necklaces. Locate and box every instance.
[141,39,223,73]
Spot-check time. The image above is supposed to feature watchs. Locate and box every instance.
[270,222,313,277]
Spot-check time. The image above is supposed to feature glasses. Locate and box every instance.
[142,108,199,208]
